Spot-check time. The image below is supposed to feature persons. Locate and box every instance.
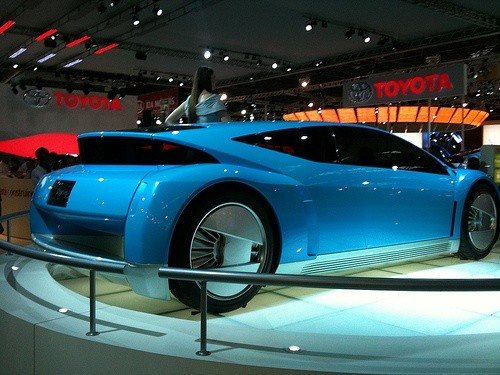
[164,66,232,126]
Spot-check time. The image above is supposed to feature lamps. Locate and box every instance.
[131,15,140,26]
[359,31,371,43]
[152,4,163,16]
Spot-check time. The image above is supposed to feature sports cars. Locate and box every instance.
[29,122,500,315]
